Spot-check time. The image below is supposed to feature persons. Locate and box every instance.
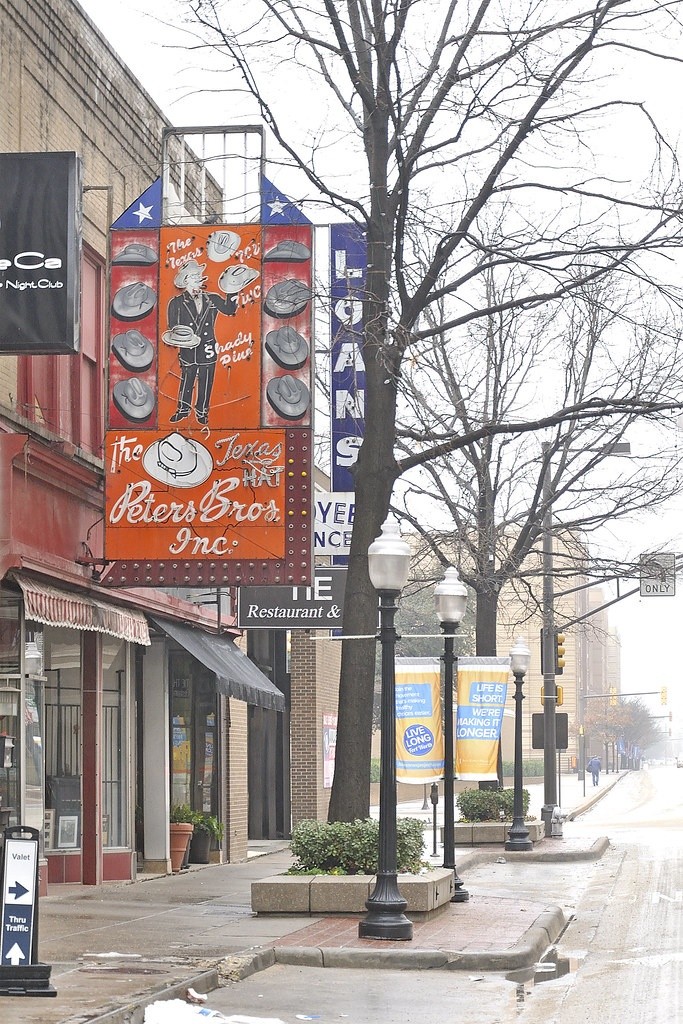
[588,754,601,786]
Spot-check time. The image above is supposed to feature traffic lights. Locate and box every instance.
[557,632,565,668]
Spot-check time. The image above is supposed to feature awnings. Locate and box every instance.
[150,616,284,713]
[13,573,152,647]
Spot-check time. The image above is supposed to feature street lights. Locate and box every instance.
[541,441,631,838]
[505,636,533,852]
[358,521,414,942]
[433,566,470,902]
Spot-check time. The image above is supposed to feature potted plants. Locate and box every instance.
[170,801,225,872]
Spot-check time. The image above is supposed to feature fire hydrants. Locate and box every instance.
[550,807,568,838]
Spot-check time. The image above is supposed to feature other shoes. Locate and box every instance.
[593,784,595,786]
[596,782,598,785]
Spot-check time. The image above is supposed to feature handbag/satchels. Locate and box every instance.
[586,766,592,772]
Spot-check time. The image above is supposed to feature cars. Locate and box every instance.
[661,758,675,765]
[677,760,683,768]
[648,759,656,766]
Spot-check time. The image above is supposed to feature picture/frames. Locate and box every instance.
[45,808,56,849]
[55,811,81,849]
